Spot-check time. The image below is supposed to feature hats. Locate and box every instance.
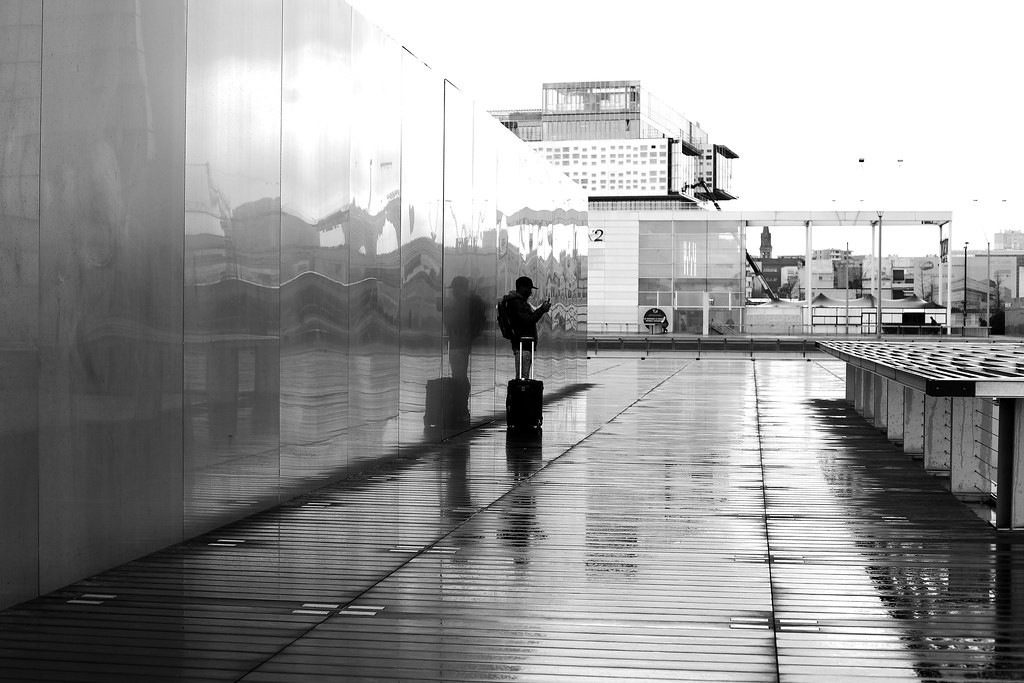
[445,276,469,288]
[516,276,539,289]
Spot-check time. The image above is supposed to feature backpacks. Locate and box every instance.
[497,295,528,340]
[466,296,487,338]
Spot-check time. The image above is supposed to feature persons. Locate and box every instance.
[437,277,473,378]
[510,276,551,379]
[930,317,938,326]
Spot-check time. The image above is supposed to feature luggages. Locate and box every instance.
[424,339,471,441]
[506,336,543,427]
